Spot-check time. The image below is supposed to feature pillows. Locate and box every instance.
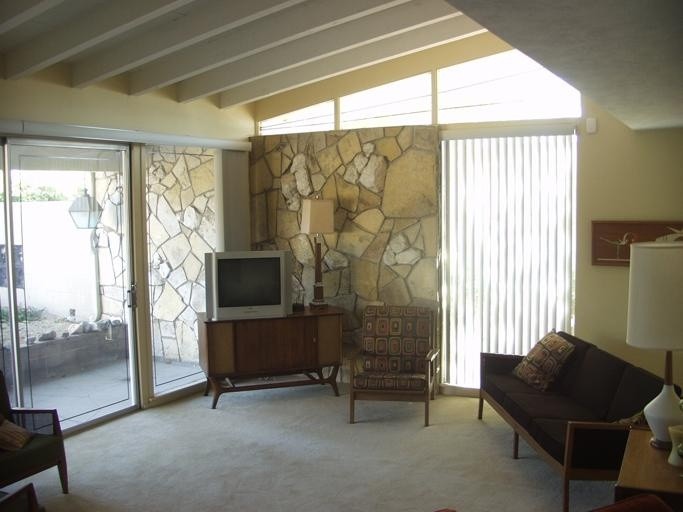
[512,332,576,394]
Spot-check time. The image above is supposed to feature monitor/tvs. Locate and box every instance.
[205,250,293,321]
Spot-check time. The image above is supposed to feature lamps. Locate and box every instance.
[298,192,335,308]
[67,188,109,230]
[626,238,681,450]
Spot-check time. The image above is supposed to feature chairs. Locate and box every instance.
[348,300,441,427]
[0,368,69,495]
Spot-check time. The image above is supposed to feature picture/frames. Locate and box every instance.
[589,218,683,267]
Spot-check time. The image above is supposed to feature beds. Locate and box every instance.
[476,330,682,512]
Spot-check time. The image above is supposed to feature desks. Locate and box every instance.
[197,310,347,409]
[600,427,683,506]
